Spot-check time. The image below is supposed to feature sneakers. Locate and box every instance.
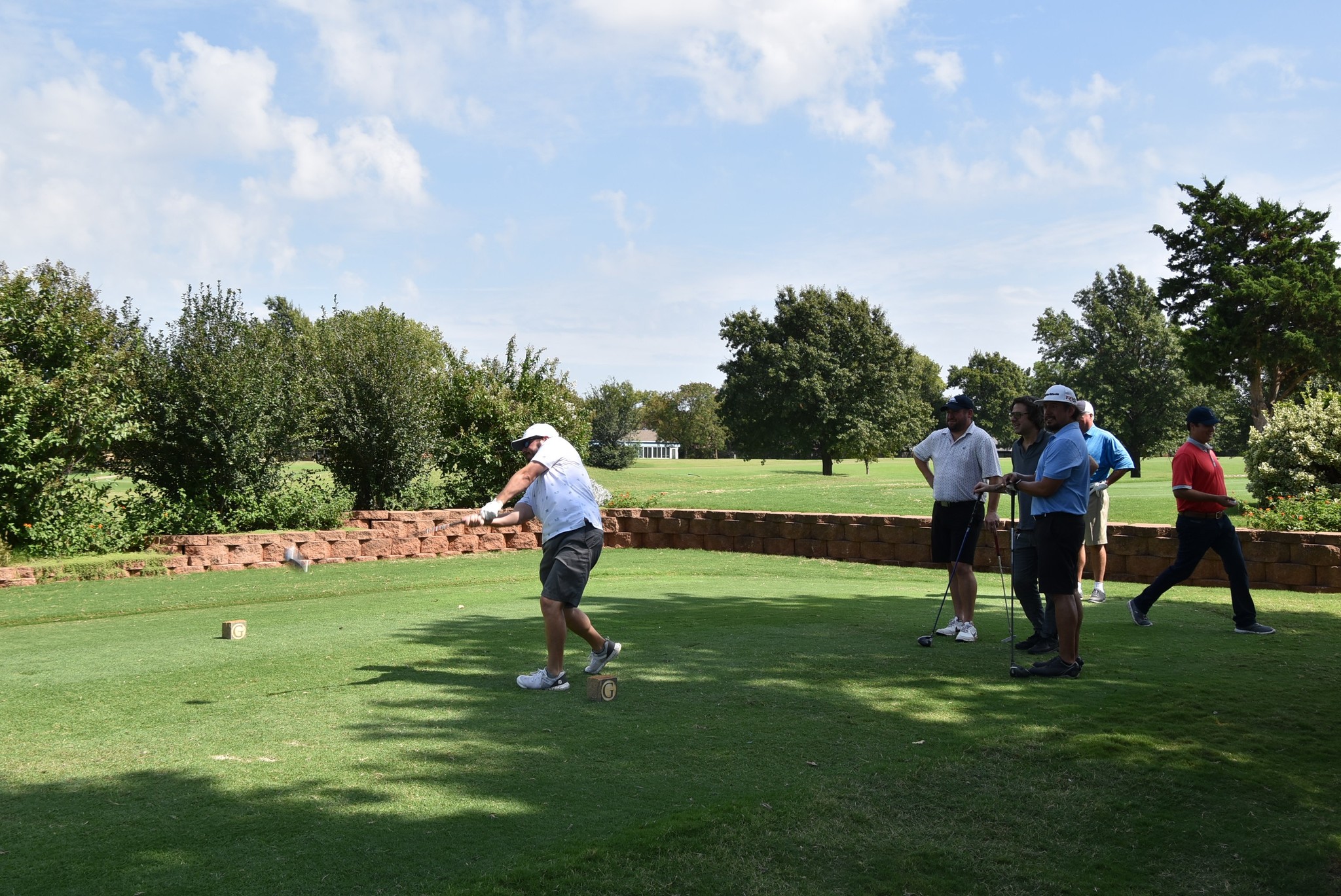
[517,667,570,691]
[1079,590,1084,600]
[936,617,963,636]
[1028,656,1084,679]
[1088,588,1106,604]
[1126,600,1153,627]
[585,637,621,674]
[956,621,977,642]
[1235,623,1276,634]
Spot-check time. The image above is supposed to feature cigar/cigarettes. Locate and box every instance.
[995,481,1010,490]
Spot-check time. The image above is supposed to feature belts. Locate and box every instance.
[935,500,984,507]
[1178,510,1224,519]
[1033,511,1083,521]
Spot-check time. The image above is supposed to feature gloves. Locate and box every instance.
[1005,484,1017,496]
[480,498,503,521]
[1090,479,1108,498]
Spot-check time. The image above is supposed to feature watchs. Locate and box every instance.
[1013,478,1022,490]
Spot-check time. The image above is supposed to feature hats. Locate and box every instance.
[1078,400,1094,415]
[940,394,973,412]
[1034,384,1078,406]
[1186,406,1223,426]
[511,423,559,452]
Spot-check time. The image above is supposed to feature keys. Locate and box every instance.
[1014,530,1021,540]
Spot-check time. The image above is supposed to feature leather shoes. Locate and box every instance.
[1027,636,1059,655]
[1015,634,1042,650]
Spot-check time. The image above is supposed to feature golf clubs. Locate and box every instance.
[1007,490,1032,680]
[916,491,982,647]
[281,517,464,575]
[991,524,1016,642]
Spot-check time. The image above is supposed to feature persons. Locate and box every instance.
[973,384,1090,678]
[912,396,1002,643]
[1076,399,1134,603]
[1126,406,1275,634]
[460,423,621,692]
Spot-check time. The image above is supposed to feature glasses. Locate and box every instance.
[1043,405,1066,410]
[1009,411,1034,420]
[517,436,543,450]
